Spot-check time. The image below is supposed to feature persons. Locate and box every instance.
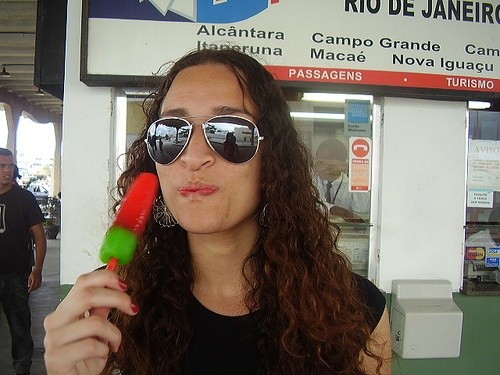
[44,50,392,375]
[316,139,371,224]
[0,148,47,375]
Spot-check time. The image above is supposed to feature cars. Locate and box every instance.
[25,183,53,204]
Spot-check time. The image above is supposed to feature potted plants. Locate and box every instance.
[40,196,61,240]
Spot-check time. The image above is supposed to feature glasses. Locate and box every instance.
[141,115,267,166]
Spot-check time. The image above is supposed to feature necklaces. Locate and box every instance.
[320,178,344,203]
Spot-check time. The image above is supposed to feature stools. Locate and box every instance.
[390,279,463,359]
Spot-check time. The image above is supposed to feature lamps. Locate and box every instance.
[0,66,10,78]
[34,88,45,96]
